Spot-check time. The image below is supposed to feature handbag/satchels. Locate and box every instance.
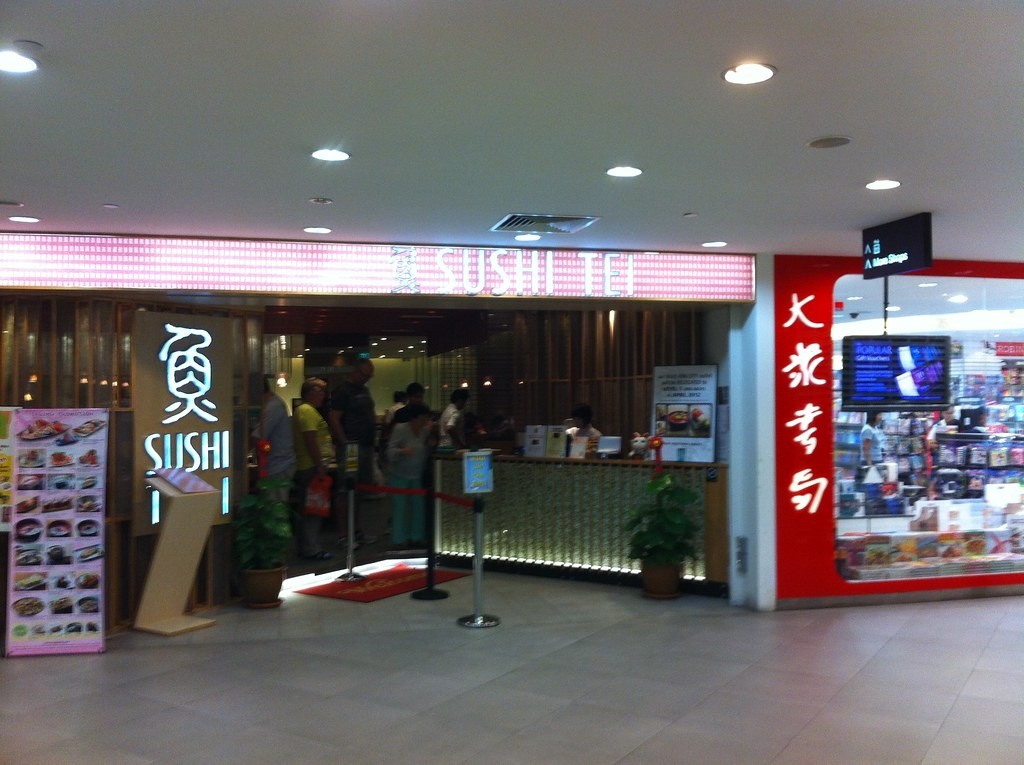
[303,475,333,516]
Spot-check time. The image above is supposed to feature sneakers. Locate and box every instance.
[358,535,378,544]
[336,536,360,550]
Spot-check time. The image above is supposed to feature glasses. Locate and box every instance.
[359,367,375,378]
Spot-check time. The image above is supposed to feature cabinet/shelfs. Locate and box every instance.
[832,395,1024,500]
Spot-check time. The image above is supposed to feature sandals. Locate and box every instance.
[311,550,331,561]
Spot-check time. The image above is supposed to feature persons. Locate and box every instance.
[565,403,603,457]
[293,375,338,560]
[327,356,380,552]
[905,403,1019,500]
[246,371,299,568]
[379,381,517,556]
[859,409,884,501]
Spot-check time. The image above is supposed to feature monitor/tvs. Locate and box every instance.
[841,336,953,412]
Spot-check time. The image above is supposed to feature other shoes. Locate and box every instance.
[392,540,411,550]
[413,539,428,549]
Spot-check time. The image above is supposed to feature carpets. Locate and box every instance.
[297,567,473,607]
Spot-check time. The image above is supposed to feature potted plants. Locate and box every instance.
[621,472,707,600]
[230,475,300,600]
